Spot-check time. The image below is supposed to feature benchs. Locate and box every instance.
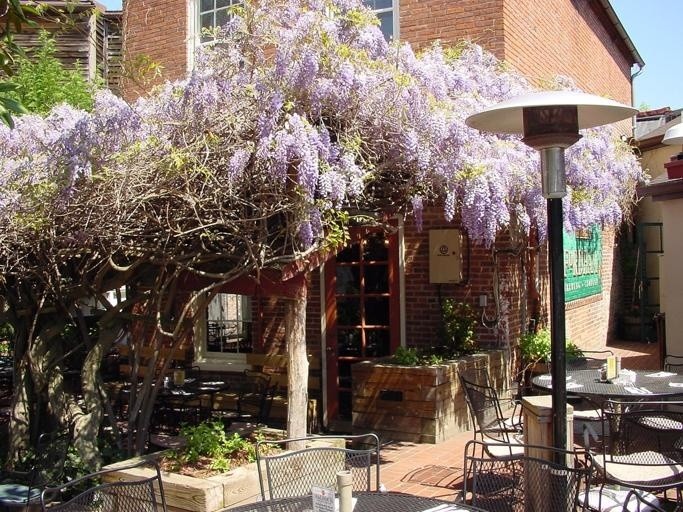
[102,345,320,431]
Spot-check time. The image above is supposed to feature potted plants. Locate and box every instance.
[517,326,588,394]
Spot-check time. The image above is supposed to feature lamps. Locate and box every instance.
[463,88,642,511]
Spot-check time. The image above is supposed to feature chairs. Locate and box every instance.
[455,348,683,512]
